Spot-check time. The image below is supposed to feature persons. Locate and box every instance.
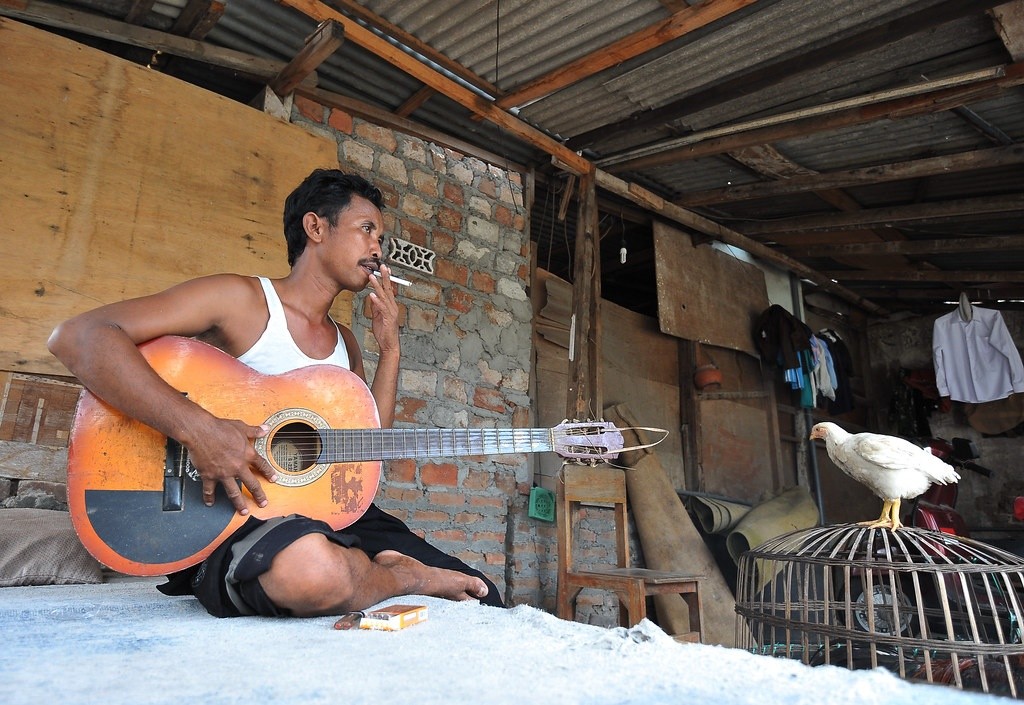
[45,166,507,622]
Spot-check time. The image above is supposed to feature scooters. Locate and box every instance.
[835,435,1024,669]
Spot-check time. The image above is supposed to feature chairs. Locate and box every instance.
[556,462,705,646]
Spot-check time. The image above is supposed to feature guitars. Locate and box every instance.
[68,334,624,578]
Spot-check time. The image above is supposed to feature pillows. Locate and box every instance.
[0,507,106,587]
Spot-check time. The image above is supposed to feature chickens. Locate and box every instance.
[810,422,961,532]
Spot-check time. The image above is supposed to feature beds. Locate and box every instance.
[1,574,1024,705]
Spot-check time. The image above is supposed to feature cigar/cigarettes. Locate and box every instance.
[356,605,430,632]
[373,270,412,288]
